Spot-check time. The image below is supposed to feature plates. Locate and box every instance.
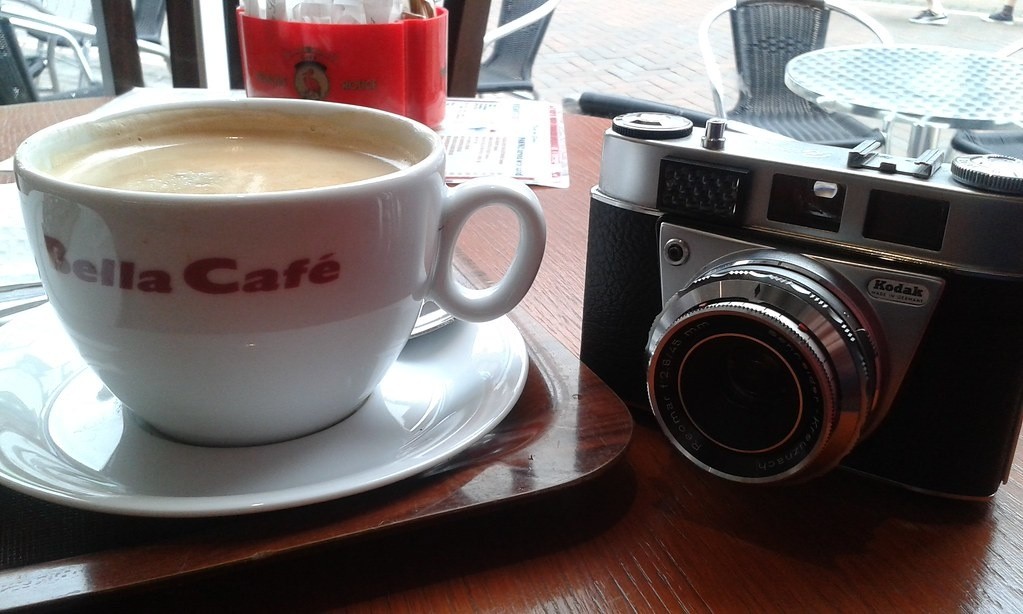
[0,300,529,517]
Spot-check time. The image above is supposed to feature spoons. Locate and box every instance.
[410,297,458,342]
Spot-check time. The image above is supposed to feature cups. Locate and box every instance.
[15,97,546,446]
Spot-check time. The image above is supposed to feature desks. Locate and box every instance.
[784,43,1023,156]
[0,93,1023,614]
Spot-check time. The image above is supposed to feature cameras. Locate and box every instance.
[581,111,1022,501]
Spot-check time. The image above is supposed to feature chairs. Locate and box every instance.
[2,0,172,91]
[695,0,895,151]
[475,0,561,102]
